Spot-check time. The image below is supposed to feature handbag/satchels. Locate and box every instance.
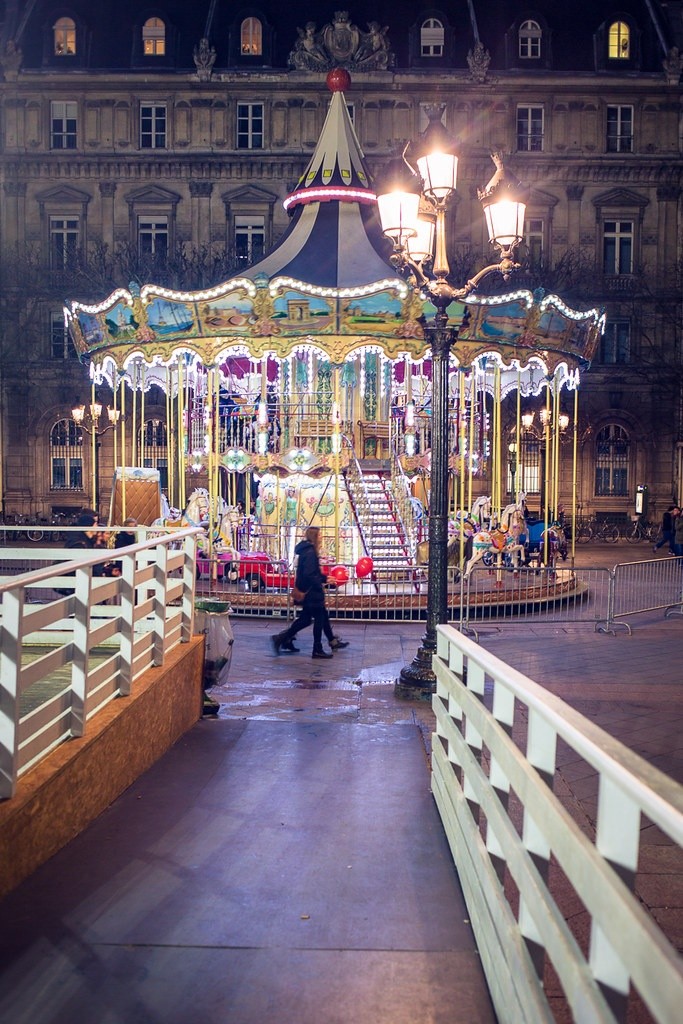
[291,587,305,601]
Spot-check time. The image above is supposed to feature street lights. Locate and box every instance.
[72,391,121,529]
[521,404,568,522]
[378,101,533,692]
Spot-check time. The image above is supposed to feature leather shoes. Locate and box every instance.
[313,644,333,658]
[329,637,350,652]
[271,634,284,655]
[281,637,300,651]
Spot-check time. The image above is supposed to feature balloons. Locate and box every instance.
[356,557,373,578]
[330,565,349,586]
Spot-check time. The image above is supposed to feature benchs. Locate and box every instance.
[294,420,352,454]
[518,519,552,551]
[358,420,393,459]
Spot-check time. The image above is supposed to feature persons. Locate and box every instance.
[213,388,239,432]
[115,518,137,605]
[271,527,350,659]
[542,505,552,523]
[63,508,120,619]
[252,385,281,435]
[652,506,683,568]
[238,500,255,513]
[355,21,387,63]
[298,22,330,64]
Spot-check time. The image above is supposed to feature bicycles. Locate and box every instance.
[560,503,664,544]
[0,507,79,543]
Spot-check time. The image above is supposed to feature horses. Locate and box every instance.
[80,486,568,582]
